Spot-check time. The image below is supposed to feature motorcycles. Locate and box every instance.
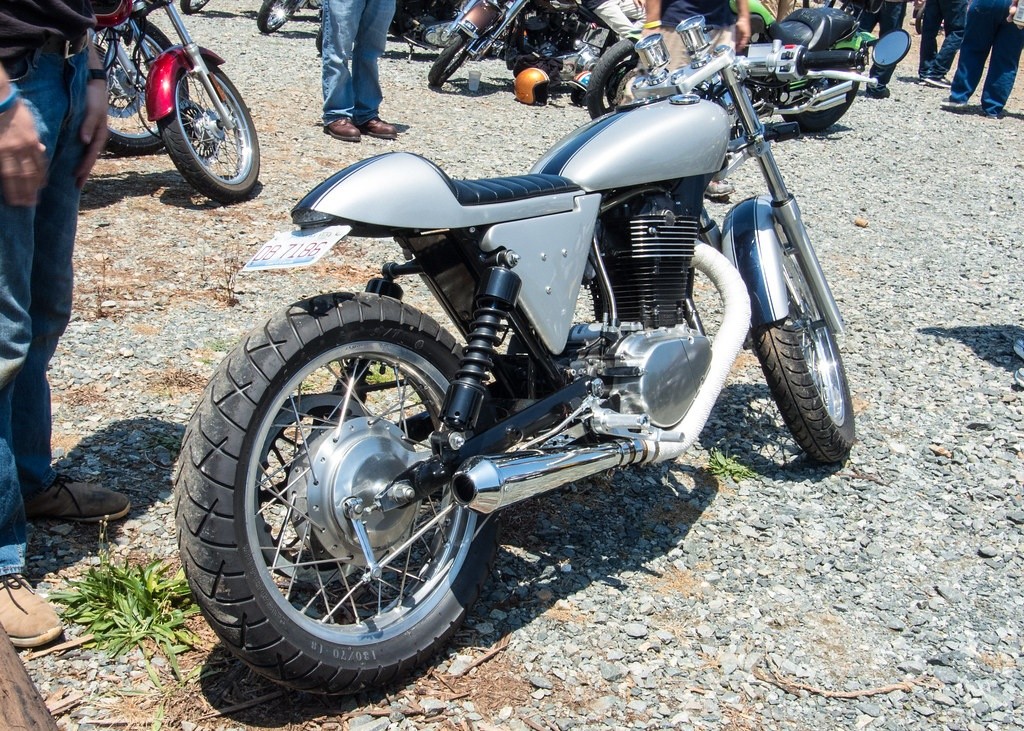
[262,1,875,135]
[167,16,912,690]
[90,0,267,202]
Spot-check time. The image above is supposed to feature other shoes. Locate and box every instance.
[924,76,953,88]
[939,97,967,110]
[867,87,890,99]
[917,77,926,86]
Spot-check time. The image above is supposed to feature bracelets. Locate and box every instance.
[89,69,107,80]
[0,84,17,114]
[643,20,662,29]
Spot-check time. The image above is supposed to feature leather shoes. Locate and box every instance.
[357,116,397,139]
[323,119,361,142]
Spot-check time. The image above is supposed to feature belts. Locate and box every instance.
[47,33,93,59]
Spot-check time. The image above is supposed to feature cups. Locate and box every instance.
[468,71,481,90]
[1013,0,1024,26]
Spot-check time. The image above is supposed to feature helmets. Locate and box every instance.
[565,71,593,107]
[514,67,551,105]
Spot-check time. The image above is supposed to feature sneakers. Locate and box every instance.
[24,472,131,522]
[0,576,63,647]
[704,180,735,198]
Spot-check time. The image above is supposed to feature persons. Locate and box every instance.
[918,0,969,89]
[322,0,397,140]
[580,0,645,41]
[0,0,131,644]
[641,0,751,197]
[940,0,1024,119]
[855,0,916,98]
